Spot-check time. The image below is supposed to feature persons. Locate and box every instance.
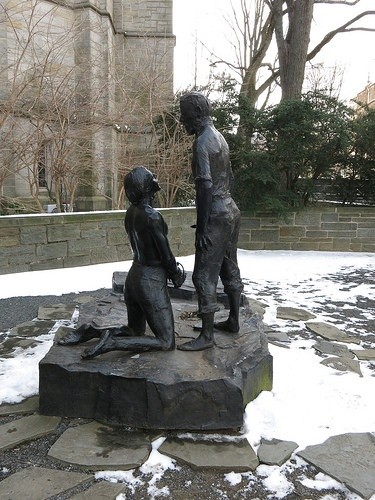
[177,91,244,351]
[58,166,187,361]
